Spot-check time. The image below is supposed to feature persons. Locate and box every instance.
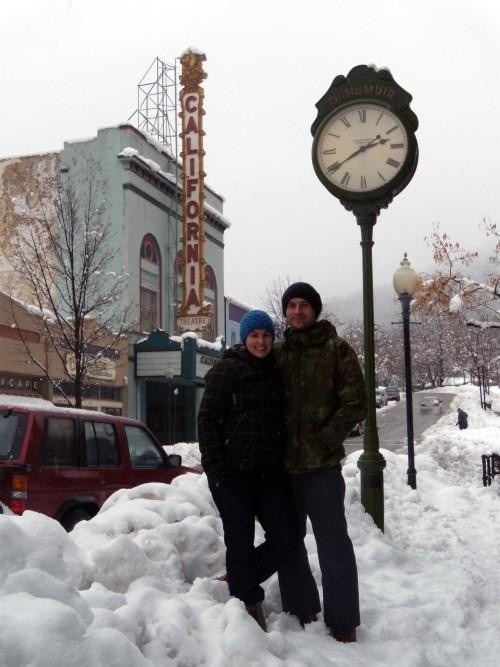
[197,308,306,636]
[268,281,370,640]
[455,408,469,430]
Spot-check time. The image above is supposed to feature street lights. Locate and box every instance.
[391,252,422,489]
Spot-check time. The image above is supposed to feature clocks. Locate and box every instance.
[311,63,418,225]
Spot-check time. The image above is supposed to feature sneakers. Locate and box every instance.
[298,606,319,628]
[330,625,356,643]
[247,601,267,631]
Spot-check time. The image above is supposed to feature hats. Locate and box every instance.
[241,310,275,346]
[282,282,323,319]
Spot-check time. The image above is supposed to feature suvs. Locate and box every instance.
[0,403,204,534]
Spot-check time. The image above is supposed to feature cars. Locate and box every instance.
[419,396,443,415]
[375,386,400,408]
